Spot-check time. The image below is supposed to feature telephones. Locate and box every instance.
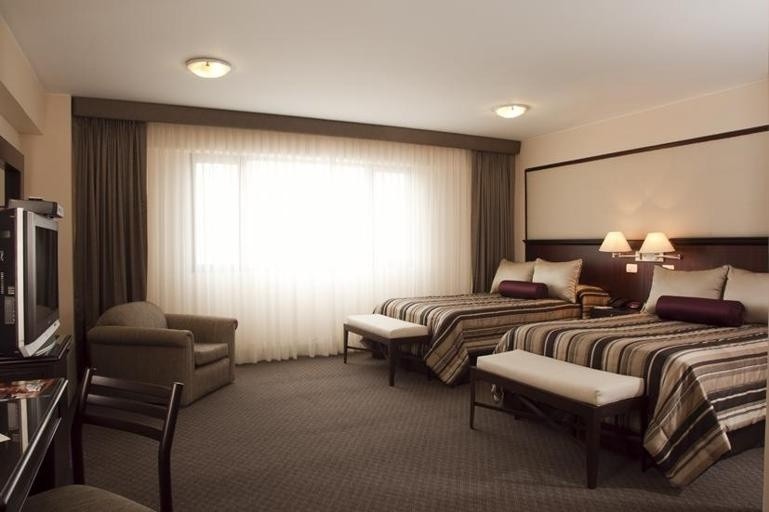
[608,296,628,309]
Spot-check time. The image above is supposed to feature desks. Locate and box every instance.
[1,377,78,512]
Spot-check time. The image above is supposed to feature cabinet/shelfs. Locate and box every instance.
[0,332,76,488]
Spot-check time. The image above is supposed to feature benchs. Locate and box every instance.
[343,314,433,386]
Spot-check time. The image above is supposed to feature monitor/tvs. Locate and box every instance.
[0,208,61,360]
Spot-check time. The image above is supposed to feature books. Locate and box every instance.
[0,377,54,403]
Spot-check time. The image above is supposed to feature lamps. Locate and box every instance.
[493,101,531,122]
[186,55,232,80]
[596,231,683,262]
